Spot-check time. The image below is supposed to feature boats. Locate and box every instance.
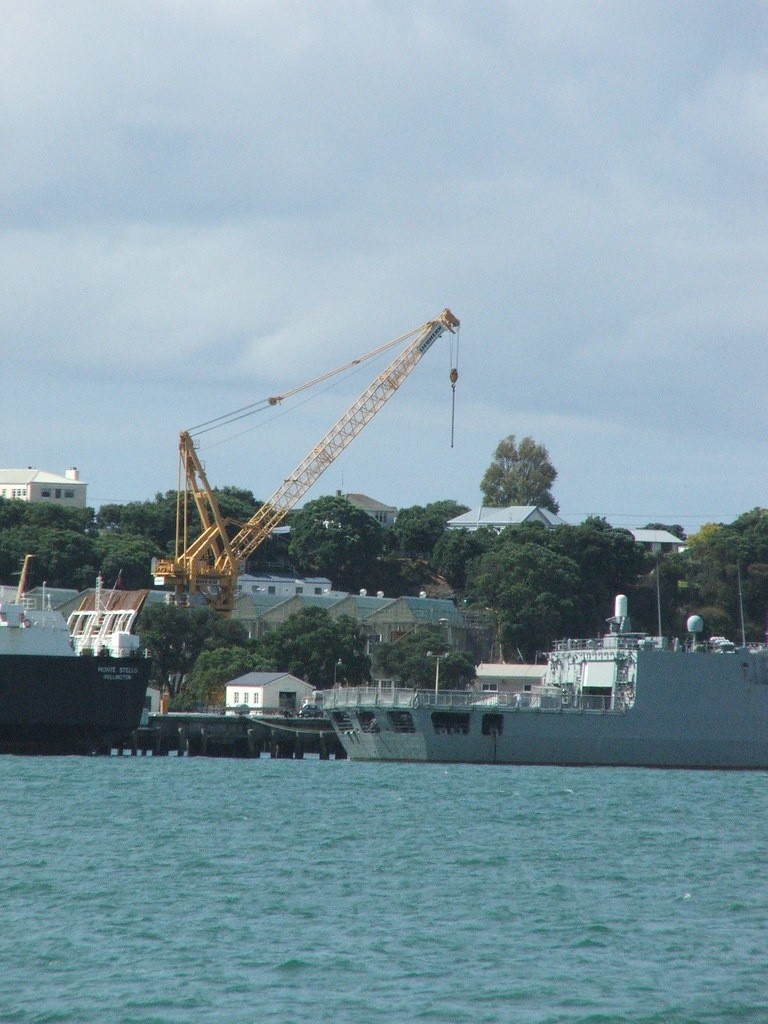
[302,561,768,771]
[0,568,153,756]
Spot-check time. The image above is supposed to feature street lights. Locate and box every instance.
[427,651,451,705]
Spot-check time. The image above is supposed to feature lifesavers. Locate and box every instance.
[23,619,31,628]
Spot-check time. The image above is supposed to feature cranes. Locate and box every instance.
[148,307,462,705]
